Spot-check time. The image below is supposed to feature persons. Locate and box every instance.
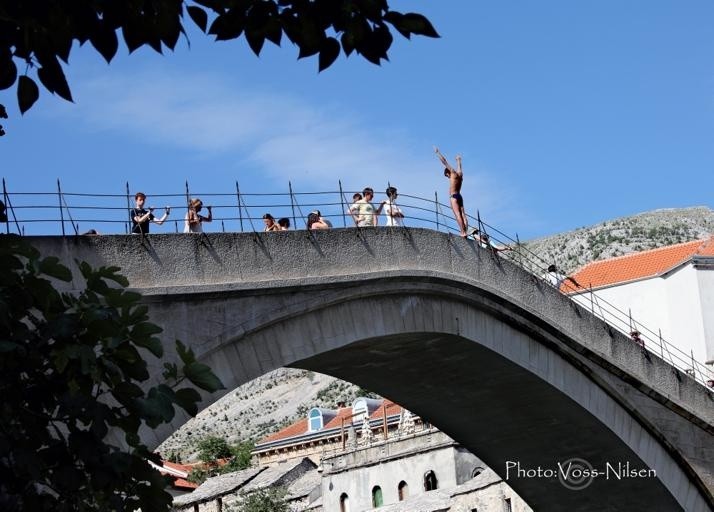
[707,380,714,389]
[279,217,290,231]
[348,188,385,227]
[383,187,405,225]
[538,265,580,292]
[313,210,332,228]
[130,192,170,234]
[353,194,361,227]
[305,213,327,229]
[261,213,281,231]
[471,230,513,254]
[184,198,212,233]
[434,146,469,240]
[629,327,644,347]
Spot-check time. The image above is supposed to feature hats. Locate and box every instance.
[468,226,478,236]
